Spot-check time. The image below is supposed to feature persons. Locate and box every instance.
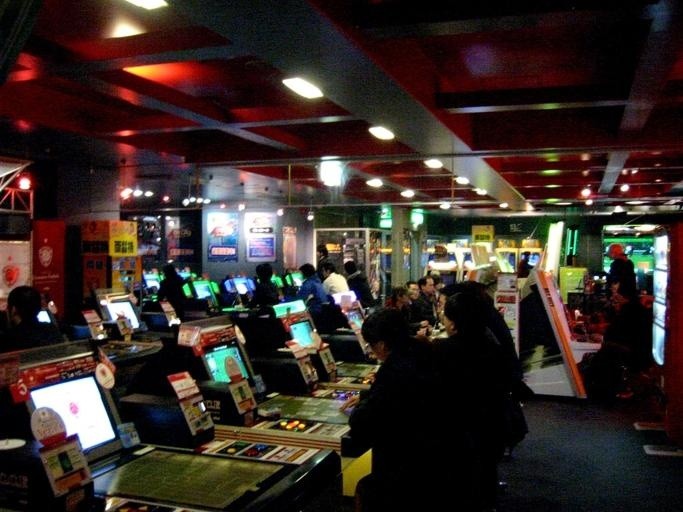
[594,252,654,369]
[440,283,515,349]
[247,263,282,309]
[157,264,189,317]
[1,285,66,349]
[341,307,471,512]
[294,244,444,326]
[518,251,532,279]
[432,289,525,473]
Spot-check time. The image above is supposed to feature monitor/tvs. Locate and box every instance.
[429,252,456,263]
[232,278,253,297]
[107,301,140,328]
[145,272,160,290]
[421,252,430,268]
[289,320,314,348]
[602,236,655,276]
[30,375,117,452]
[384,253,406,269]
[205,346,250,385]
[500,252,515,271]
[270,274,276,283]
[193,281,219,307]
[36,311,51,323]
[159,301,181,327]
[177,272,191,280]
[291,273,304,287]
[460,252,473,267]
[346,308,364,329]
[521,253,539,266]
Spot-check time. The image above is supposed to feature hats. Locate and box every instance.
[603,244,628,257]
